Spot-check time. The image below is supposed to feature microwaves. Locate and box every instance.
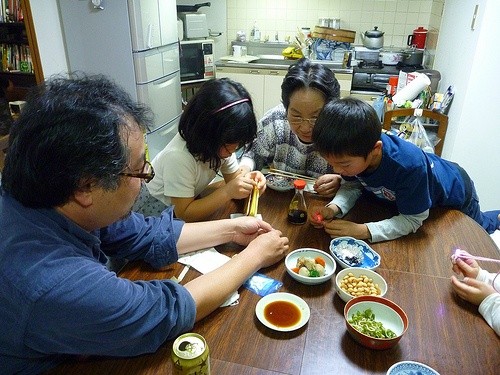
[180,39,217,84]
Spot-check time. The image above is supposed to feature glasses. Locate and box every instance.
[283,107,318,126]
[115,160,156,183]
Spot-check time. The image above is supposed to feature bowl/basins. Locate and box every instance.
[329,236,382,271]
[265,172,297,191]
[335,267,388,302]
[344,295,409,349]
[285,248,336,285]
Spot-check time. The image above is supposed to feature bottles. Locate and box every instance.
[288,180,308,224]
[254,28,261,40]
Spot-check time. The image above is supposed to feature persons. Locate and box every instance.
[449,250,500,338]
[128,76,266,222]
[311,96,500,245]
[0,70,289,375]
[240,62,341,197]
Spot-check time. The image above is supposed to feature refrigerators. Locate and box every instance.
[59,0,184,162]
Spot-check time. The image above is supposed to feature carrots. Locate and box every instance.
[291,268,300,274]
[315,258,325,266]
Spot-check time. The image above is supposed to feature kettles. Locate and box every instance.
[360,26,385,49]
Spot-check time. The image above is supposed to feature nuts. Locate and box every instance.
[340,273,381,297]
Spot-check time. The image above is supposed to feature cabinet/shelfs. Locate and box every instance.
[216,68,290,124]
[0,0,47,98]
[383,91,450,158]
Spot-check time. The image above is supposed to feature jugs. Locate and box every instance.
[407,27,429,52]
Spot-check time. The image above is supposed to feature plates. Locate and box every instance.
[255,292,311,332]
[386,361,441,375]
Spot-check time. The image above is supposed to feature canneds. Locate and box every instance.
[171,333,211,375]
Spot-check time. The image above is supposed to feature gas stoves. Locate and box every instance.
[351,45,441,93]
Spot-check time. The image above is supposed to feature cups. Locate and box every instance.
[318,16,340,29]
[232,45,241,57]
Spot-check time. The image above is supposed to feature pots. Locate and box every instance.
[176,2,210,12]
[402,44,424,66]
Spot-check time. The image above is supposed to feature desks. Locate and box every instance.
[180,78,210,109]
[46,177,500,375]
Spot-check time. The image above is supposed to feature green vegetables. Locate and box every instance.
[349,307,397,338]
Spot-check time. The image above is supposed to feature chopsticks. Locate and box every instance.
[268,168,316,182]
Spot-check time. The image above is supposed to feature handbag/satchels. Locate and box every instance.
[404,118,434,153]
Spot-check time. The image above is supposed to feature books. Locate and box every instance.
[0,43,35,73]
[0,0,24,23]
